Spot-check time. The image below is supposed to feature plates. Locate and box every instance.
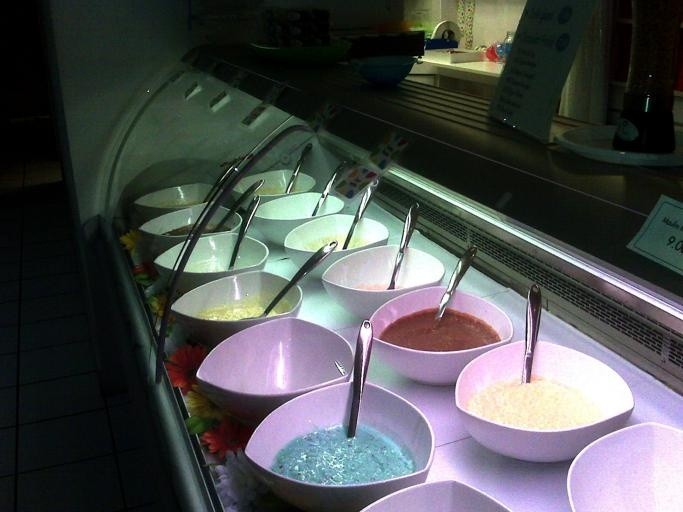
[553,125,683,165]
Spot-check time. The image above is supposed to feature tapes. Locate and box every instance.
[430,20,461,44]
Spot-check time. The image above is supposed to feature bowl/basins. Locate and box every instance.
[171,270,305,340]
[153,232,270,295]
[565,422,682,512]
[195,316,354,421]
[243,379,435,512]
[369,286,514,388]
[250,192,345,247]
[134,181,223,221]
[230,169,316,211]
[138,201,242,254]
[352,56,415,87]
[453,340,635,464]
[323,244,446,320]
[247,39,352,70]
[285,214,390,281]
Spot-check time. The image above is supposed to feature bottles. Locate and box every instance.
[609,1,682,153]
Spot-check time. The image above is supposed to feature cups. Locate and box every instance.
[496,32,514,62]
